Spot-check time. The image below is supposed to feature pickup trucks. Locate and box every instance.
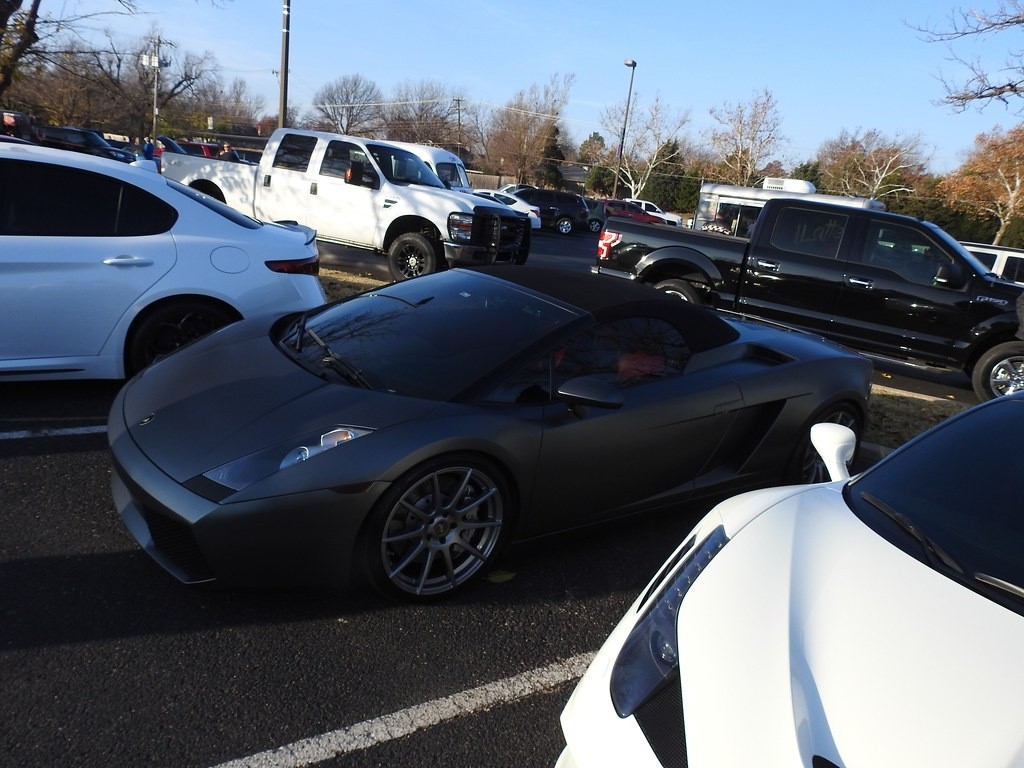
[160,127,531,282]
[591,198,1023,403]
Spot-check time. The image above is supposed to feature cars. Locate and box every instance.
[107,260,885,603]
[1,143,328,393]
[1,109,263,170]
[958,241,1023,288]
[552,388,1023,768]
[472,183,685,238]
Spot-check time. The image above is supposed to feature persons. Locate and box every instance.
[220,143,240,163]
